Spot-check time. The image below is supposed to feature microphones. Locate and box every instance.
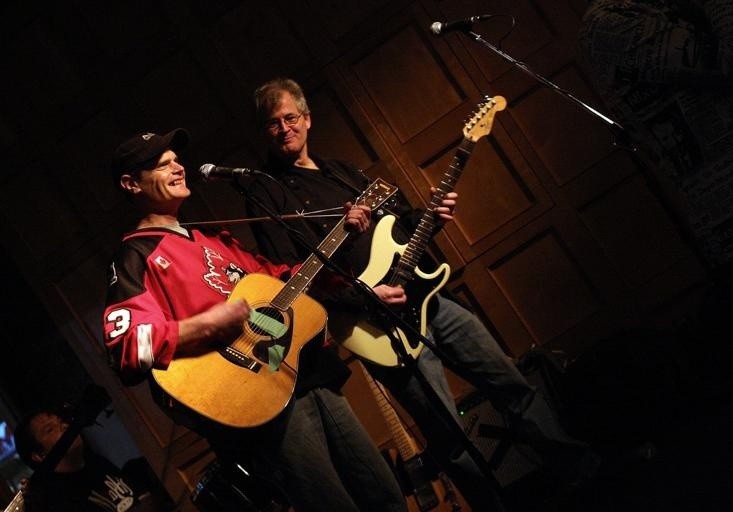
[198,161,260,184]
[430,13,485,37]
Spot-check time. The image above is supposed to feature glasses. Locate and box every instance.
[260,110,304,129]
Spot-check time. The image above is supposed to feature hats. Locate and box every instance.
[106,128,192,178]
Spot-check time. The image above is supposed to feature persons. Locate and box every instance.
[579,0,733,301]
[104,78,603,511]
[15,410,141,512]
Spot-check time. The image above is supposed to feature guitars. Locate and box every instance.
[328,94,507,370]
[147,177,399,442]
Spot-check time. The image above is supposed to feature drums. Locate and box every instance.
[190,461,293,511]
